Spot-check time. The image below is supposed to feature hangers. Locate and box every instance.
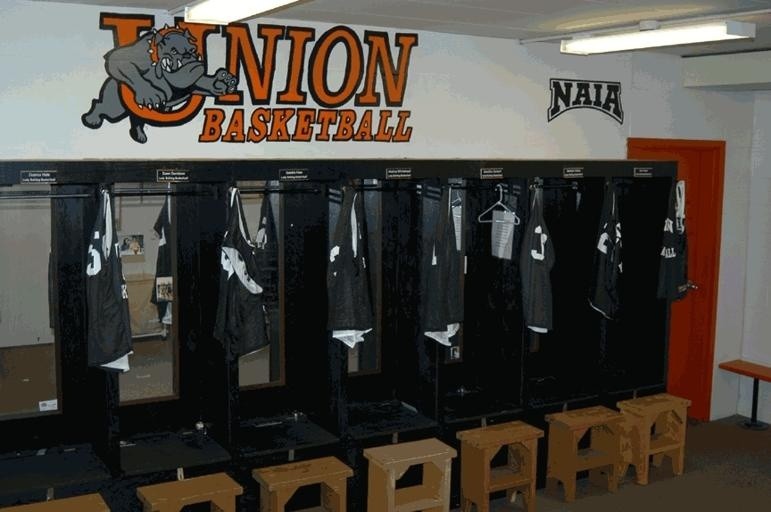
[477,182,522,228]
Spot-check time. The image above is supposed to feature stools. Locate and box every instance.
[456,421,543,511]
[363,439,455,511]
[2,493,108,511]
[543,406,619,502]
[251,457,354,511]
[618,393,691,485]
[135,473,244,511]
